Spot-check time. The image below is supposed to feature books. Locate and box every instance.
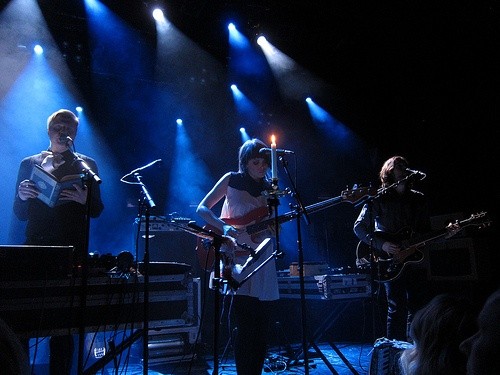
[27,162,86,211]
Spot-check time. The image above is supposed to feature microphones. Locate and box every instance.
[406,168,426,175]
[129,157,163,176]
[259,147,295,156]
[59,134,72,142]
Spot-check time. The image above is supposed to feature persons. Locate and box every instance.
[13,109,104,375]
[400,291,500,375]
[195,138,283,375]
[353,155,461,341]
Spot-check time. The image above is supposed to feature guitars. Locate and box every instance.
[355,208,495,283]
[194,179,381,273]
